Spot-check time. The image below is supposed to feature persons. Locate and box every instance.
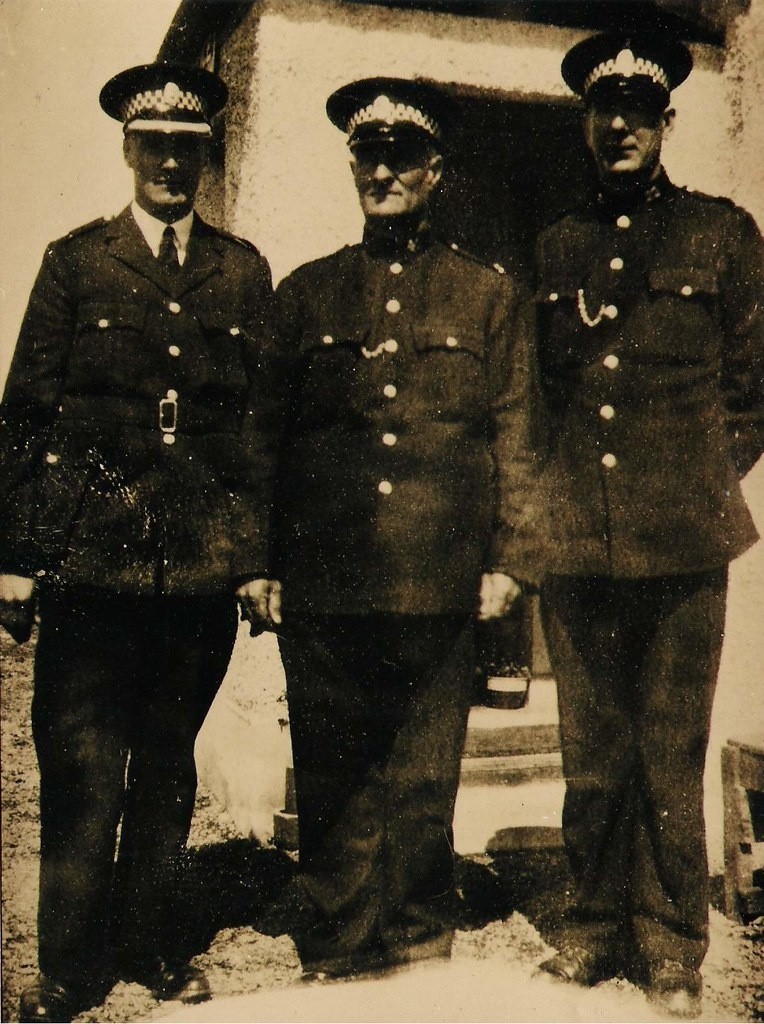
[270,67,528,980]
[0,60,280,1021]
[509,23,764,1016]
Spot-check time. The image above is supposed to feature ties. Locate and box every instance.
[157,225,181,285]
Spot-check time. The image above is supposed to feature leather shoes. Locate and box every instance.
[18,963,120,1022]
[537,943,623,985]
[121,951,212,1005]
[625,942,704,1017]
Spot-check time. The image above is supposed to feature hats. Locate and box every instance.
[560,27,693,105]
[98,63,230,137]
[324,76,459,147]
